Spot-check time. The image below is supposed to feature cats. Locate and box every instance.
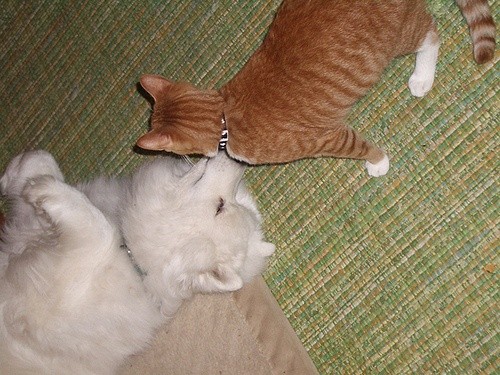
[136,0,500,177]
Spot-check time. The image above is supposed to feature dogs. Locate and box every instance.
[0,149,277,375]
